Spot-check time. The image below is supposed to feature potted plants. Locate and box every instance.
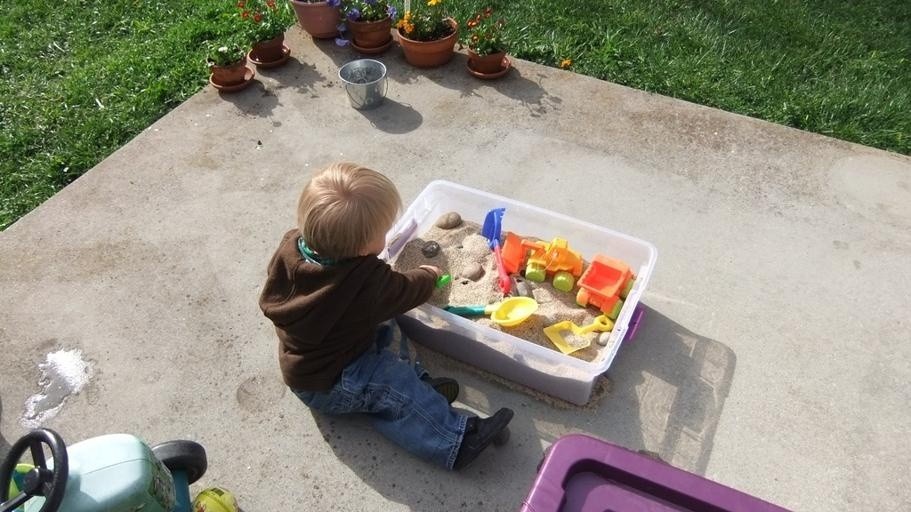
[205,14,247,83]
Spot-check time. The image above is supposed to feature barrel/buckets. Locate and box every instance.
[339,58,389,111]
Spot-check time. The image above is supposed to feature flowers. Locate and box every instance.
[395,0,459,42]
[324,0,398,48]
[236,0,297,43]
[465,4,516,57]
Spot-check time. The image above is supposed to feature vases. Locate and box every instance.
[348,15,390,49]
[467,44,506,74]
[252,34,284,62]
[396,16,459,68]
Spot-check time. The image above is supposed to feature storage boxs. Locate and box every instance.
[519,432,795,511]
[379,179,658,407]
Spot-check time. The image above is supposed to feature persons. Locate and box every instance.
[260,161,513,468]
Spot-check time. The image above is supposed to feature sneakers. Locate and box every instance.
[453,408,514,471]
[420,372,459,404]
